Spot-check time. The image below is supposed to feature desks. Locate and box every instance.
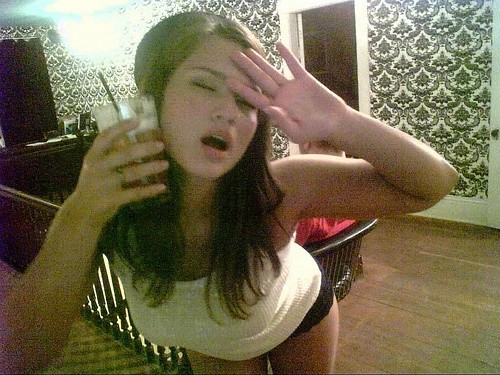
[0,127,99,184]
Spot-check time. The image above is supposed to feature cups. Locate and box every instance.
[93,97,172,213]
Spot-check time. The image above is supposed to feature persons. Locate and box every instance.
[294,140,359,246]
[0,12,458,373]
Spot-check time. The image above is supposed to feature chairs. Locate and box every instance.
[303,218,381,303]
